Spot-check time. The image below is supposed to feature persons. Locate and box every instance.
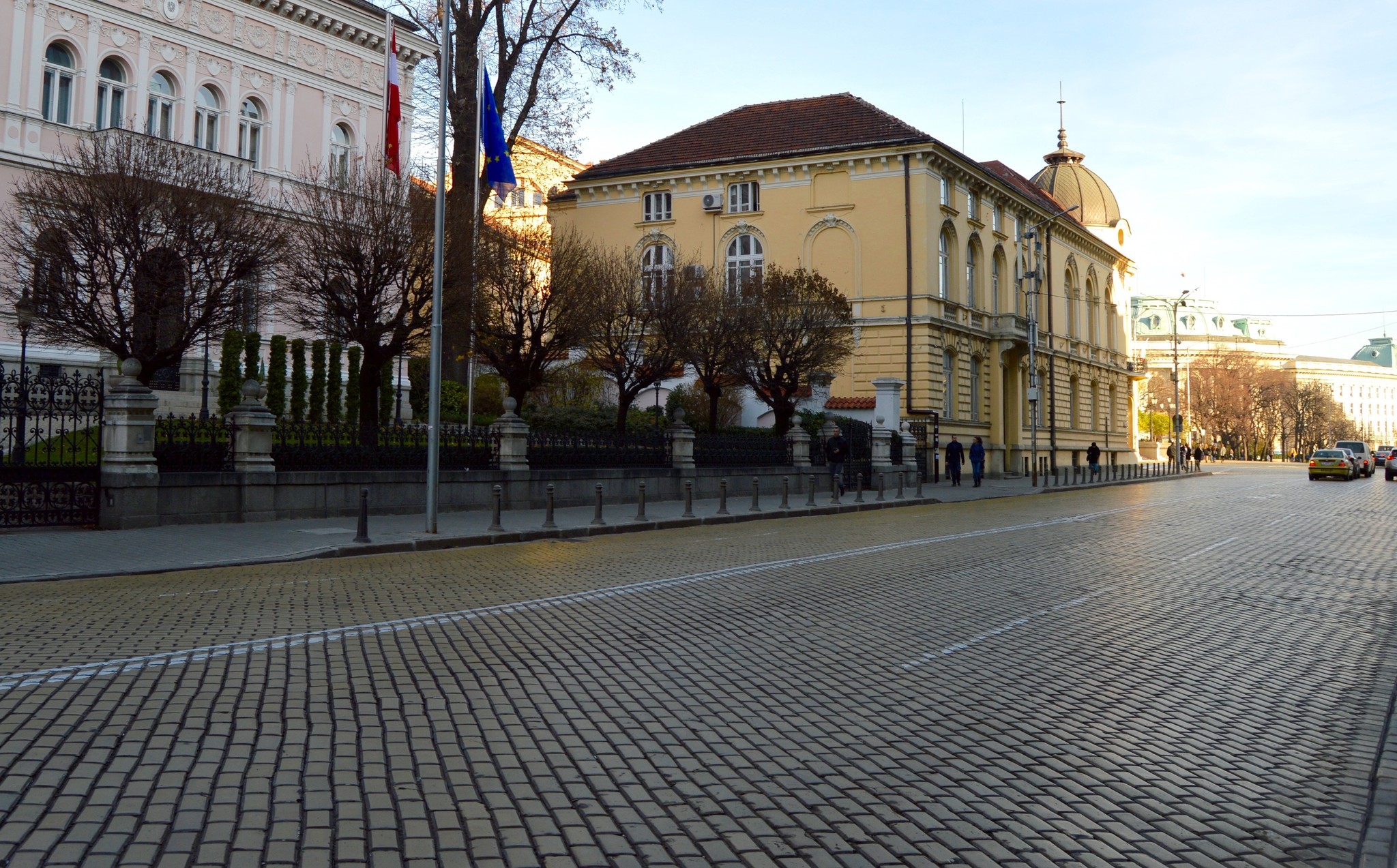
[1087,442,1100,475]
[945,434,965,488]
[1229,447,1297,462]
[969,437,985,487]
[1167,441,1226,472]
[825,426,847,499]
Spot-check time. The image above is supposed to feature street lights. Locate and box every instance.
[1020,204,1079,487]
[10,286,37,462]
[199,301,213,418]
[1141,288,1199,474]
[653,377,660,432]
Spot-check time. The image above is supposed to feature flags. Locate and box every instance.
[481,61,517,203]
[384,22,402,178]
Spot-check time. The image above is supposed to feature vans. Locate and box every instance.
[1332,440,1376,478]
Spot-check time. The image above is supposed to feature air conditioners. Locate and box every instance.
[702,194,723,209]
[684,264,703,279]
[686,286,701,300]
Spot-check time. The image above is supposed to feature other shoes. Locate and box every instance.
[978,484,981,487]
[1094,471,1097,475]
[957,481,961,486]
[832,494,834,497]
[841,485,846,496]
[952,484,956,487]
[973,485,978,487]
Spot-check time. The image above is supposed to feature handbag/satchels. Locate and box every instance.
[1086,455,1090,461]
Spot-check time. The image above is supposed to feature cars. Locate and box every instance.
[1372,445,1395,464]
[1332,447,1360,479]
[1308,449,1355,481]
[1384,448,1397,480]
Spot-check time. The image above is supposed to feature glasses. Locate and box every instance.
[953,437,957,439]
[973,439,977,441]
